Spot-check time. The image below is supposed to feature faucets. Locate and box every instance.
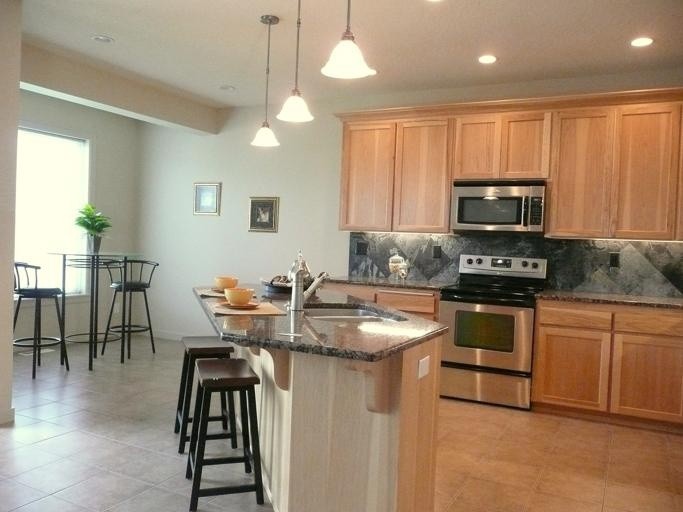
[290,270,329,311]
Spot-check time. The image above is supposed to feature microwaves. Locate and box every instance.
[448,177,545,233]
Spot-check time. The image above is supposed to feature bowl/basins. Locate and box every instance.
[223,315,254,332]
[214,275,239,292]
[223,288,254,306]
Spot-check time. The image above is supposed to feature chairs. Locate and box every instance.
[101,260,159,354]
[12,263,71,379]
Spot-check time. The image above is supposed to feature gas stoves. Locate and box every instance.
[439,282,541,308]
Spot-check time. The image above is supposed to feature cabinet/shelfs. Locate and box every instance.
[545,85,683,243]
[334,104,454,232]
[324,274,458,324]
[531,289,683,435]
[453,96,552,180]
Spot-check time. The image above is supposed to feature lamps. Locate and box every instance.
[276,0,314,124]
[250,16,280,147]
[321,0,377,80]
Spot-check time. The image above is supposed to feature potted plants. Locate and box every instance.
[76,203,112,253]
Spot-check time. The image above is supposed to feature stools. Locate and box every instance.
[186,359,264,512]
[174,337,238,455]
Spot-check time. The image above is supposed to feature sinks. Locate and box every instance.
[303,308,398,323]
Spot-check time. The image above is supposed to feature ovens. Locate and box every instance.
[436,294,535,413]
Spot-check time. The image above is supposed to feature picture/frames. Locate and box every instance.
[248,197,278,232]
[192,182,222,216]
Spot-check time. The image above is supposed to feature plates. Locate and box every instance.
[220,303,258,309]
[212,288,224,293]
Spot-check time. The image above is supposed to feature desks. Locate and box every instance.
[48,250,144,364]
